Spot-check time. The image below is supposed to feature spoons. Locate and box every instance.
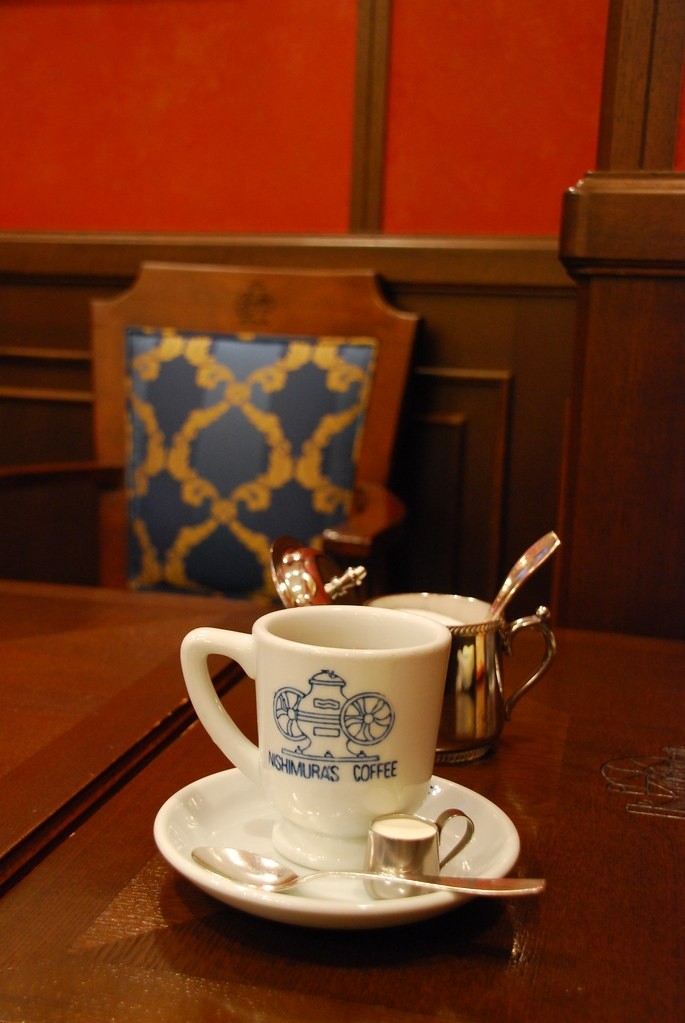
[191,846,546,897]
[270,534,332,608]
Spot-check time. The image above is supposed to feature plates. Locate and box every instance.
[153,768,520,928]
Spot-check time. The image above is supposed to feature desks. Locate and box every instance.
[0,578,685,1023]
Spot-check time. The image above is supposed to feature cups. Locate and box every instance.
[365,808,475,900]
[180,605,451,872]
[366,593,558,764]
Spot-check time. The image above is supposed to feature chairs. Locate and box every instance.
[87,266,428,605]
